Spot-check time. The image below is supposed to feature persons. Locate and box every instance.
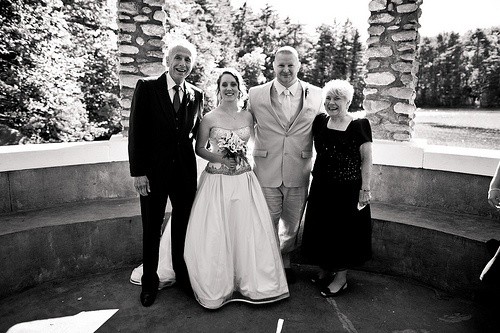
[220,106,238,120]
[127,38,204,307]
[488,159,500,209]
[247,46,329,285]
[298,79,373,297]
[130,67,290,309]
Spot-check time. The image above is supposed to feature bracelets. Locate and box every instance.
[361,188,371,193]
[487,188,500,192]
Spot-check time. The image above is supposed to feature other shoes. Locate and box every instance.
[285,268,296,284]
[140,287,157,306]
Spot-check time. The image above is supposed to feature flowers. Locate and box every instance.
[218,130,250,171]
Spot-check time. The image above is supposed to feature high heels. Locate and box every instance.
[320,281,348,297]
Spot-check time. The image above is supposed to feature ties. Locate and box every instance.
[281,90,291,128]
[172,85,181,113]
